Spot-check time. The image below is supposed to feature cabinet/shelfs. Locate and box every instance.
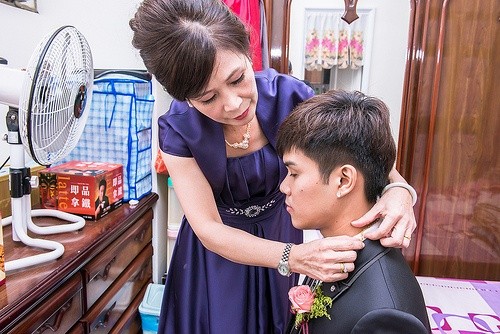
[0,192,159,334]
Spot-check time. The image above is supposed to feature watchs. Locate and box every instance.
[276,241,296,277]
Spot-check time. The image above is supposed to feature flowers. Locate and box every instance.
[287,283,332,334]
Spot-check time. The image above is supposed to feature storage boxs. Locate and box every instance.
[38,160,125,221]
[139,283,166,334]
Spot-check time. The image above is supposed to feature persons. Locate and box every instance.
[273,87,433,334]
[94,179,110,219]
[127,0,419,334]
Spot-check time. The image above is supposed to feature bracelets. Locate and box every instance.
[379,181,418,207]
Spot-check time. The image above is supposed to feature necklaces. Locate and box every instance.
[225,120,252,152]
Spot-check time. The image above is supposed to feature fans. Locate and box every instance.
[0,24,95,272]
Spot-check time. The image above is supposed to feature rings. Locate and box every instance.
[404,235,412,241]
[339,263,348,273]
